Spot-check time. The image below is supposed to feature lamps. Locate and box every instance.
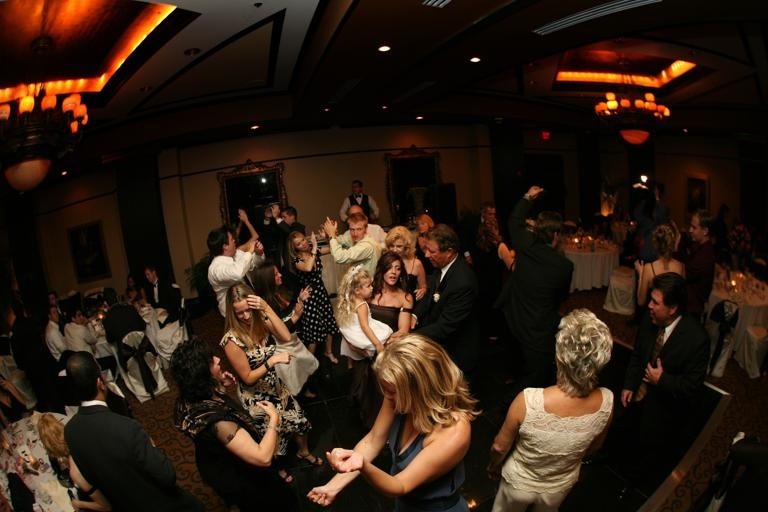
[0,39,90,195]
[593,53,672,146]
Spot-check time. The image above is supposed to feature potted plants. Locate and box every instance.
[184,252,217,303]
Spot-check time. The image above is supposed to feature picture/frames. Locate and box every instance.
[63,219,113,284]
[682,169,713,218]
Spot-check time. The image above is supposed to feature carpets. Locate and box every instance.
[562,336,734,512]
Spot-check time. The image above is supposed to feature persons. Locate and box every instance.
[0,173,767,512]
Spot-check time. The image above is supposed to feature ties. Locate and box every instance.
[633,327,665,403]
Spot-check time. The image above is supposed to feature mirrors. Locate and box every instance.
[215,155,291,251]
[383,143,445,226]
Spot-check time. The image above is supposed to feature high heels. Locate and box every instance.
[295,451,324,467]
[324,351,338,364]
[277,468,294,485]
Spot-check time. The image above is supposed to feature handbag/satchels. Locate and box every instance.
[264,331,320,397]
